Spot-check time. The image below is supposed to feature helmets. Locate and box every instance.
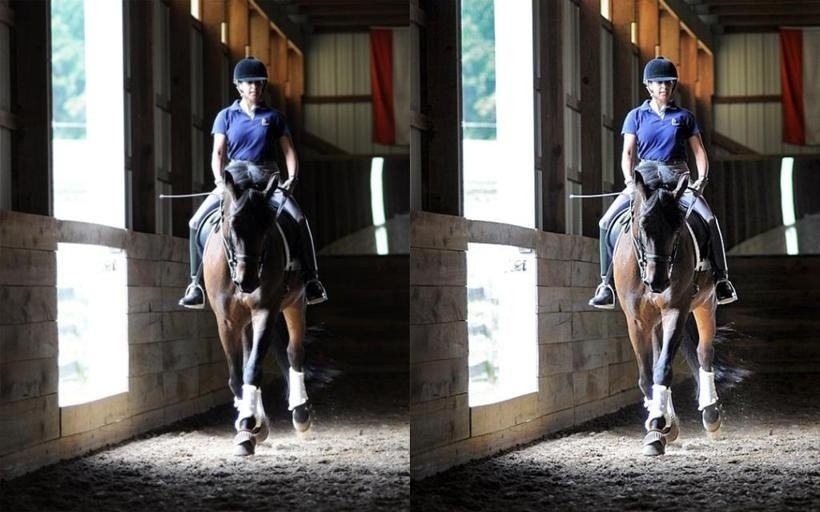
[642,57,678,82]
[233,56,267,88]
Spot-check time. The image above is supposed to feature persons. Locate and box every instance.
[178,57,329,309]
[588,57,739,305]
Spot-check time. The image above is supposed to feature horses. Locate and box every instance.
[611,168,721,458]
[201,168,312,462]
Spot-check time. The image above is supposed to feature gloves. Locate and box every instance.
[691,176,708,195]
[282,176,299,195]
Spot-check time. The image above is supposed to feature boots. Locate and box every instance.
[588,228,617,305]
[177,228,207,306]
[704,215,734,301]
[293,214,324,301]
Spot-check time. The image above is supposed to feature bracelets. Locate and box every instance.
[624,176,633,184]
[698,176,709,183]
